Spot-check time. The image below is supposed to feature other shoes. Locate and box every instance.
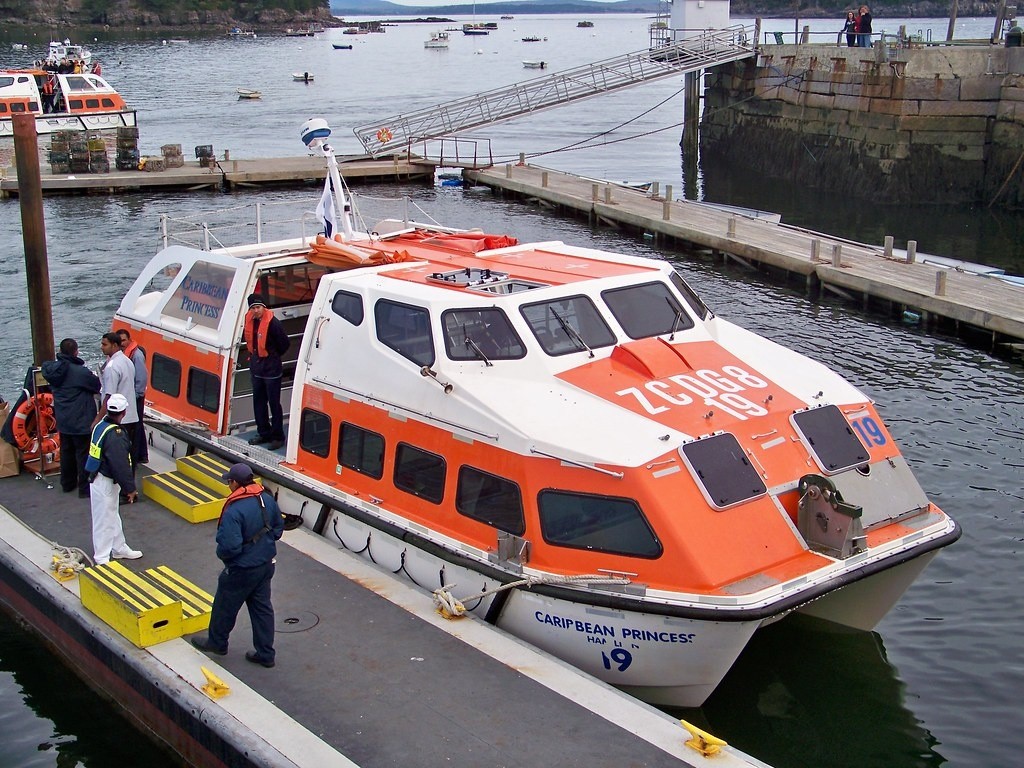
[79,492,89,497]
[249,435,272,444]
[119,495,138,506]
[112,549,142,560]
[270,439,286,452]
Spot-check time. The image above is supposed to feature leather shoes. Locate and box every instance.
[245,650,276,667]
[191,636,228,655]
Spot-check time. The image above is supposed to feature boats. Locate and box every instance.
[286,28,301,36]
[226,27,256,39]
[484,22,497,27]
[237,87,262,98]
[30,39,92,70]
[292,72,314,81]
[110,118,962,707]
[462,29,489,35]
[343,22,385,34]
[501,17,513,20]
[332,44,352,50]
[424,38,448,48]
[297,28,314,36]
[308,23,324,33]
[576,21,594,27]
[0,69,138,138]
[522,37,539,41]
[12,44,28,51]
[522,61,548,69]
[169,40,190,45]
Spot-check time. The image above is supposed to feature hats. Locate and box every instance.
[248,294,267,309]
[107,394,129,413]
[222,464,254,483]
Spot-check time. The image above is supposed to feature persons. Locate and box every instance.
[190,462,286,668]
[244,294,291,451]
[840,11,856,47]
[40,339,101,498]
[860,5,873,48]
[84,392,144,565]
[90,333,140,505]
[35,57,102,77]
[854,6,865,47]
[115,329,149,464]
[38,79,58,113]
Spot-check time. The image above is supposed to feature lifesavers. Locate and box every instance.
[377,128,393,142]
[12,393,60,454]
